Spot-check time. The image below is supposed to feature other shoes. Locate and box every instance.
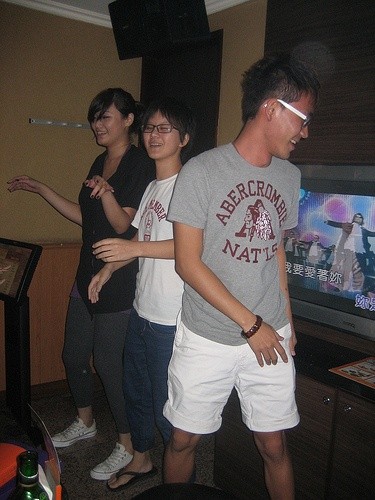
[320,286,340,292]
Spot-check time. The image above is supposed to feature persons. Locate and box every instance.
[161,54,321,500]
[7,88,156,482]
[87,94,197,491]
[282,211,375,296]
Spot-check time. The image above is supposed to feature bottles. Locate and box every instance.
[5,450,50,500]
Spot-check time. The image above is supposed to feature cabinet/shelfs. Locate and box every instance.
[213,371,375,500]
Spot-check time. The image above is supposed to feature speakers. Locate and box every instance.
[107,0,212,61]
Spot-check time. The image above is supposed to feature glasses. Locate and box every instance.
[264,98,314,129]
[143,124,179,133]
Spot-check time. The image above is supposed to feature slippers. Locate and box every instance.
[107,467,158,492]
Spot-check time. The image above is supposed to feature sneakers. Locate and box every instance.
[90,442,133,480]
[52,416,97,447]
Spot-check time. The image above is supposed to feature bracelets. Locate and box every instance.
[240,315,263,339]
